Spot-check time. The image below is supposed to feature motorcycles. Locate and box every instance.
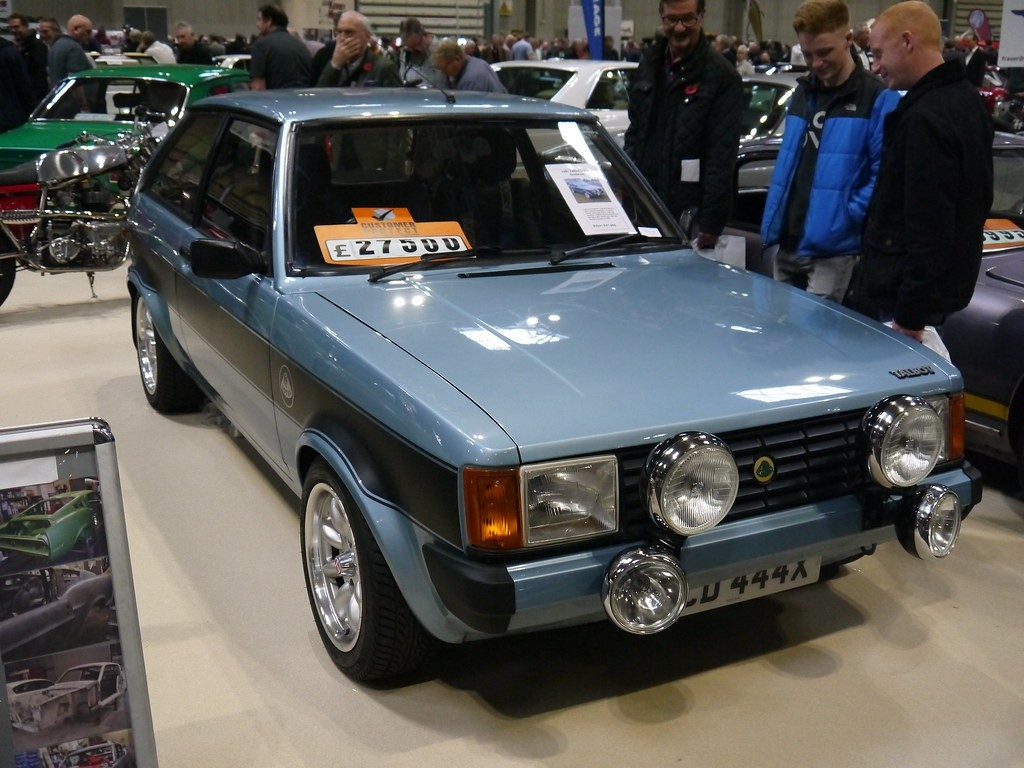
[0,105,192,307]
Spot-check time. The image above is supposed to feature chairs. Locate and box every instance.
[262,143,353,265]
[113,93,145,121]
[427,125,543,250]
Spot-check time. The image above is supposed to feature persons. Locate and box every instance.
[398,18,449,89]
[463,27,665,88]
[943,35,997,89]
[251,5,314,91]
[623,0,745,249]
[303,9,407,177]
[46,14,99,115]
[0,14,62,132]
[430,38,517,176]
[850,24,874,70]
[83,21,259,65]
[761,0,902,306]
[842,0,995,342]
[706,33,808,101]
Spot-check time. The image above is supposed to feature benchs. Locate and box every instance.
[217,179,407,222]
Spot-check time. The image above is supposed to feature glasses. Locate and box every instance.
[661,13,703,27]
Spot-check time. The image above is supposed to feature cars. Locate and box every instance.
[0,64,251,192]
[932,132,1023,499]
[736,72,809,198]
[118,86,985,691]
[488,59,642,150]
[212,52,252,69]
